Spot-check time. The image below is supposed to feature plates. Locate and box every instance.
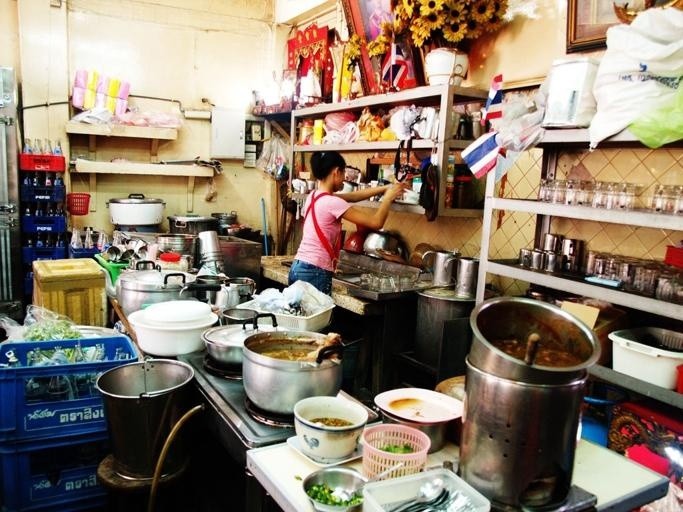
[286,432,366,467]
[372,387,463,425]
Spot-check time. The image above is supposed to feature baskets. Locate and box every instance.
[66,192,91,216]
[360,422,431,480]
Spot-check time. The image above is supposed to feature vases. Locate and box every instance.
[422,46,470,87]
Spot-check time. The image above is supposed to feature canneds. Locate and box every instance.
[445,182,454,208]
[453,176,473,208]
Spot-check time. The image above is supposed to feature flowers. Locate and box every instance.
[343,26,363,102]
[389,1,515,49]
[366,20,391,94]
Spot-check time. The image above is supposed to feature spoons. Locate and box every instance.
[388,475,452,511]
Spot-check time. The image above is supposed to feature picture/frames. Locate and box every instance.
[566,1,682,54]
[340,1,426,97]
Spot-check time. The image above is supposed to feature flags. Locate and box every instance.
[461,131,501,180]
[380,32,409,93]
[482,74,503,119]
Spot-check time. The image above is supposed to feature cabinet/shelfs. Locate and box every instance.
[473,124,682,411]
[64,119,214,215]
[288,82,486,223]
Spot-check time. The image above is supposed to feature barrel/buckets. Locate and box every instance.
[196,229,224,270]
[157,234,193,258]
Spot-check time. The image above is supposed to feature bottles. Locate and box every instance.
[456,119,482,140]
[1,343,128,406]
[69,226,121,251]
[21,137,63,159]
[584,249,683,305]
[21,172,66,278]
[297,119,314,146]
[445,154,455,210]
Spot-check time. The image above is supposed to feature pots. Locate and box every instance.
[153,210,238,269]
[411,284,495,369]
[361,230,398,260]
[105,193,166,225]
[126,300,291,365]
[194,274,227,304]
[237,314,348,418]
[224,277,254,306]
[116,260,195,318]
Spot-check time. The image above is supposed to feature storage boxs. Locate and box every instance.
[544,56,599,129]
[32,251,106,328]
[559,297,611,366]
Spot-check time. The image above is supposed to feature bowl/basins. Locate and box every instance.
[102,238,148,263]
[302,467,368,511]
[468,294,602,386]
[341,229,363,253]
[293,394,369,462]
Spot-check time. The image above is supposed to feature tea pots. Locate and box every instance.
[420,244,479,300]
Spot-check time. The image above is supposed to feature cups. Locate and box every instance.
[69,67,131,119]
[515,230,582,277]
[356,273,415,295]
[536,177,683,217]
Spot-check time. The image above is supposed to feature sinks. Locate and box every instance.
[281,260,424,302]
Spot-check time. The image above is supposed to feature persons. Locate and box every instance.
[288,150,408,298]
